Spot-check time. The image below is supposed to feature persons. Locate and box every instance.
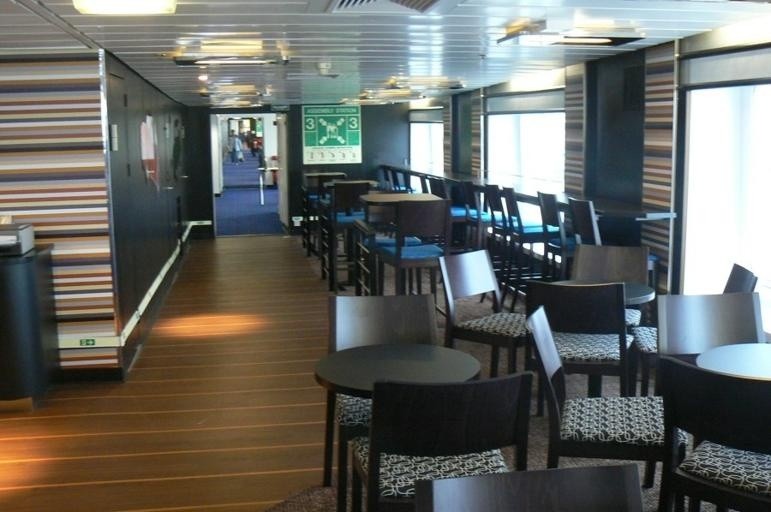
[228,130,243,166]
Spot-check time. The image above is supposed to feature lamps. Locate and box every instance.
[72,0,176,17]
[173,37,290,67]
[341,75,467,106]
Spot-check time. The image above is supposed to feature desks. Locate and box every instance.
[318,200,332,279]
[380,163,677,223]
[695,342,771,379]
[314,343,481,488]
[358,192,444,230]
[0,243,58,409]
[625,279,656,326]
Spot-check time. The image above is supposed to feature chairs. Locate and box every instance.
[571,241,650,397]
[480,182,540,311]
[415,460,644,512]
[305,173,347,257]
[360,236,423,295]
[329,181,374,292]
[328,294,437,512]
[525,305,663,488]
[500,185,561,312]
[568,195,601,249]
[437,249,535,377]
[350,371,534,512]
[376,200,452,319]
[656,291,765,355]
[533,189,571,278]
[524,280,635,418]
[461,178,516,254]
[630,264,757,397]
[428,176,484,258]
[657,355,770,512]
